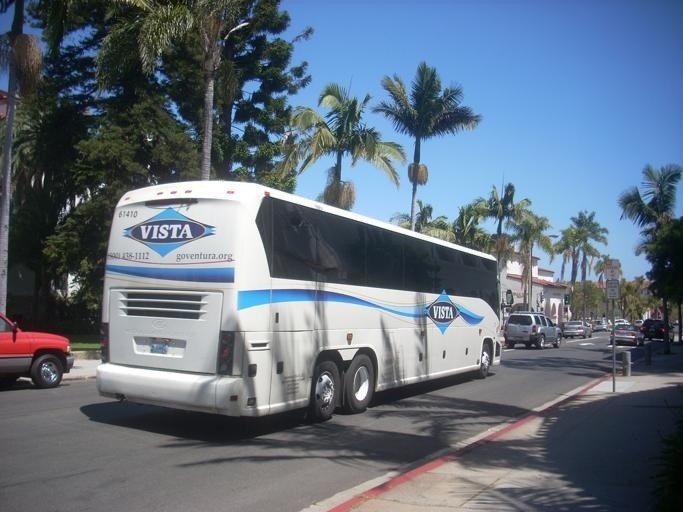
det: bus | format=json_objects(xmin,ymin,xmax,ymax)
[{"xmin": 96, "ymin": 180, "xmax": 505, "ymax": 421}]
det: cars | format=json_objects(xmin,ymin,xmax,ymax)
[
  {"xmin": 558, "ymin": 318, "xmax": 645, "ymax": 347},
  {"xmin": 0, "ymin": 312, "xmax": 74, "ymax": 389}
]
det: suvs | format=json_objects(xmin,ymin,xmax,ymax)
[
  {"xmin": 504, "ymin": 311, "xmax": 563, "ymax": 350},
  {"xmin": 640, "ymin": 319, "xmax": 675, "ymax": 343}
]
